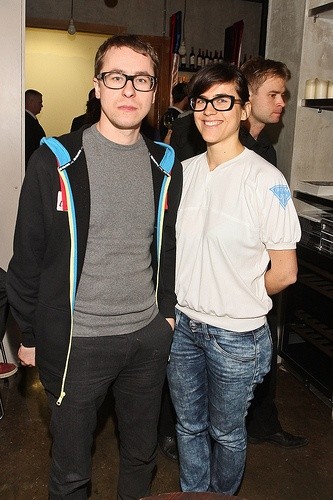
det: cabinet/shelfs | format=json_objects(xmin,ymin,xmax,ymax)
[{"xmin": 274, "ymin": 242, "xmax": 333, "ymax": 407}]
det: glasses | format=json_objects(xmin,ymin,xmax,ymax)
[
  {"xmin": 96, "ymin": 72, "xmax": 157, "ymax": 92},
  {"xmin": 188, "ymin": 95, "xmax": 242, "ymax": 112}
]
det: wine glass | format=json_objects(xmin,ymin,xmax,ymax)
[{"xmin": 163, "ymin": 113, "xmax": 174, "ymax": 132}]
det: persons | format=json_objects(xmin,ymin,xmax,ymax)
[
  {"xmin": 162, "ymin": 58, "xmax": 307, "ymax": 460},
  {"xmin": 70, "ymin": 88, "xmax": 101, "ymax": 135},
  {"xmin": 160, "ymin": 82, "xmax": 191, "ymax": 137},
  {"xmin": 7, "ymin": 36, "xmax": 182, "ymax": 500},
  {"xmin": 168, "ymin": 59, "xmax": 300, "ymax": 495},
  {"xmin": 25, "ymin": 89, "xmax": 45, "ymax": 171}
]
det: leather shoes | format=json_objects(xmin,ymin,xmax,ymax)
[
  {"xmin": 246, "ymin": 429, "xmax": 308, "ymax": 448},
  {"xmin": 158, "ymin": 433, "xmax": 179, "ymax": 463}
]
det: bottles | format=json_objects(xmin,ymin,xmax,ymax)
[
  {"xmin": 202, "ymin": 51, "xmax": 204, "ymax": 68},
  {"xmin": 205, "ymin": 49, "xmax": 209, "ymax": 66},
  {"xmin": 210, "ymin": 52, "xmax": 213, "ymax": 63},
  {"xmin": 180, "ymin": 54, "xmax": 187, "ymax": 67},
  {"xmin": 213, "ymin": 50, "xmax": 218, "ymax": 63},
  {"xmin": 190, "ymin": 47, "xmax": 195, "ymax": 69},
  {"xmin": 197, "ymin": 49, "xmax": 202, "ymax": 68},
  {"xmin": 218, "ymin": 50, "xmax": 224, "ymax": 63}
]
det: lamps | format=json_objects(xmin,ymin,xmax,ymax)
[
  {"xmin": 68, "ymin": 0, "xmax": 76, "ymax": 35},
  {"xmin": 178, "ymin": 0, "xmax": 187, "ymax": 55}
]
